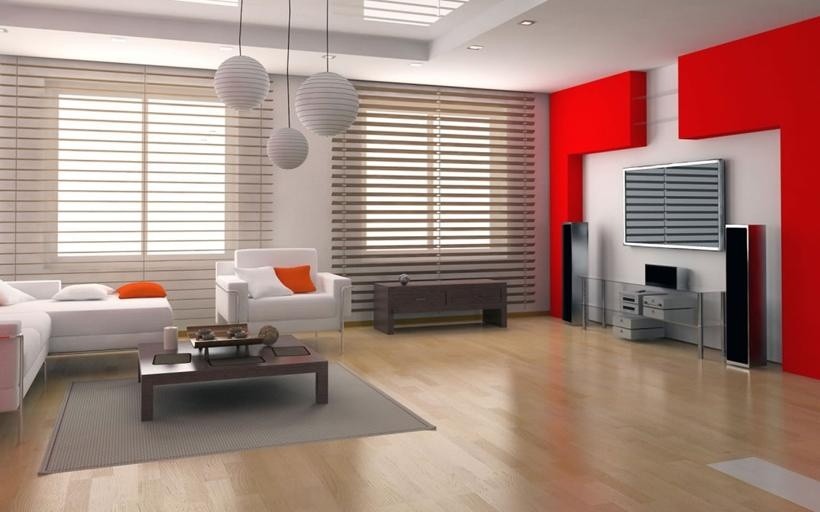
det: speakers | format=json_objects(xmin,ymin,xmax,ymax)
[
  {"xmin": 562, "ymin": 220, "xmax": 589, "ymax": 324},
  {"xmin": 721, "ymin": 224, "xmax": 767, "ymax": 370}
]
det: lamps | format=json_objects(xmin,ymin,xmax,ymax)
[
  {"xmin": 294, "ymin": 0, "xmax": 359, "ymax": 139},
  {"xmin": 213, "ymin": 0, "xmax": 270, "ymax": 113},
  {"xmin": 266, "ymin": 0, "xmax": 309, "ymax": 170}
]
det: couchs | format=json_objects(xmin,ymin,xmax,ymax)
[{"xmin": 1, "ymin": 279, "xmax": 175, "ymax": 445}]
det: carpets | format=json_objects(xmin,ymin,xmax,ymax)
[{"xmin": 36, "ymin": 360, "xmax": 437, "ymax": 477}]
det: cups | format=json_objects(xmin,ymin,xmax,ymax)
[{"xmin": 163, "ymin": 326, "xmax": 178, "ymax": 351}]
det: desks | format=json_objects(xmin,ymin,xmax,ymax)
[{"xmin": 373, "ymin": 278, "xmax": 508, "ymax": 335}]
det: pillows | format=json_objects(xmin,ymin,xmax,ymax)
[
  {"xmin": 234, "ymin": 266, "xmax": 295, "ymax": 300},
  {"xmin": 116, "ymin": 283, "xmax": 167, "ymax": 300},
  {"xmin": 233, "ymin": 246, "xmax": 318, "ymax": 296},
  {"xmin": 274, "ymin": 265, "xmax": 317, "ymax": 294},
  {"xmin": 1, "ymin": 280, "xmax": 34, "ymax": 306},
  {"xmin": 52, "ymin": 283, "xmax": 116, "ymax": 301}
]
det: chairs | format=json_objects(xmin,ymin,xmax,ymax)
[{"xmin": 214, "ymin": 247, "xmax": 352, "ymax": 355}]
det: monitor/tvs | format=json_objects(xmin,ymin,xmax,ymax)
[{"xmin": 622, "ymin": 159, "xmax": 727, "ymax": 253}]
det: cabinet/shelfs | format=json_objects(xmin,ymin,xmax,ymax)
[{"xmin": 576, "ymin": 273, "xmax": 725, "ymax": 361}]
